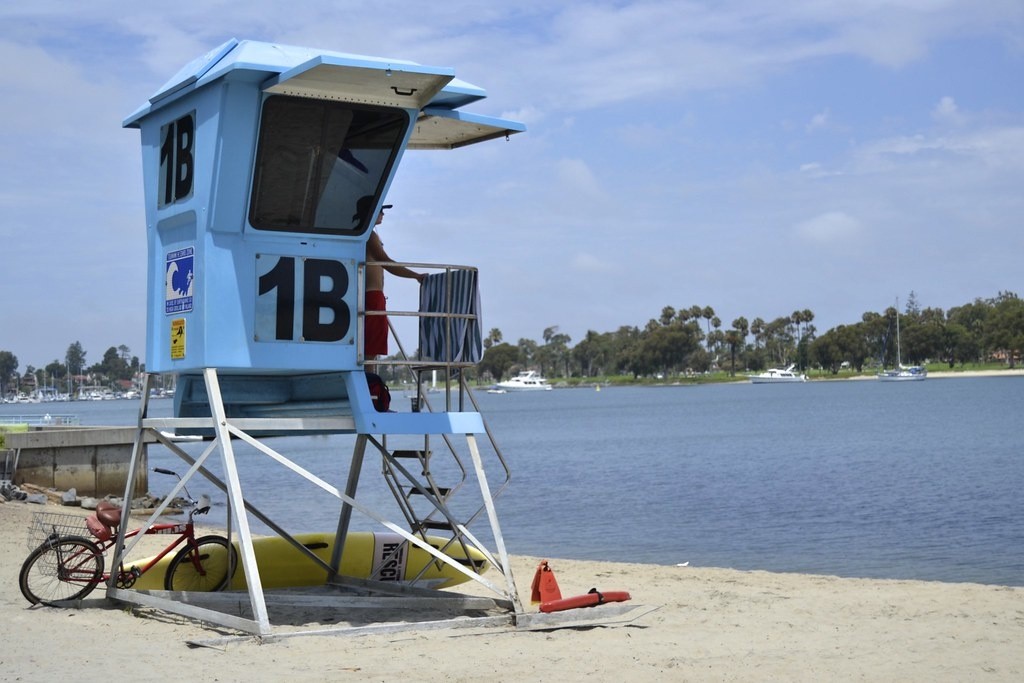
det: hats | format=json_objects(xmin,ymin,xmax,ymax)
[{"xmin": 357, "ymin": 195, "xmax": 393, "ymax": 213}]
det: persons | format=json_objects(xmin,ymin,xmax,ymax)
[{"xmin": 352, "ymin": 196, "xmax": 429, "ymax": 373}]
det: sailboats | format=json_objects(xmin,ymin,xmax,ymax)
[
  {"xmin": 876, "ymin": 296, "xmax": 927, "ymax": 381},
  {"xmin": 0, "ymin": 366, "xmax": 175, "ymax": 405}
]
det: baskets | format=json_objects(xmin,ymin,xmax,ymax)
[
  {"xmin": 27, "ymin": 513, "xmax": 90, "ymax": 553},
  {"xmin": 37, "ymin": 532, "xmax": 107, "ymax": 579}
]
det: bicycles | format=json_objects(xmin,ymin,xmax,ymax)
[{"xmin": 19, "ymin": 468, "xmax": 237, "ymax": 608}]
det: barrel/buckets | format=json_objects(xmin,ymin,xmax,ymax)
[{"xmin": 411, "ymin": 398, "xmax": 419, "ymax": 412}]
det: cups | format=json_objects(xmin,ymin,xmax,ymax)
[{"xmin": 197, "ymin": 494, "xmax": 210, "ymax": 510}]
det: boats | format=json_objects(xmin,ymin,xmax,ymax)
[
  {"xmin": 427, "ymin": 388, "xmax": 439, "ymax": 394},
  {"xmin": 749, "ymin": 369, "xmax": 805, "ymax": 385},
  {"xmin": 487, "ymin": 389, "xmax": 506, "ymax": 394},
  {"xmin": 496, "ymin": 370, "xmax": 553, "ymax": 391}
]
